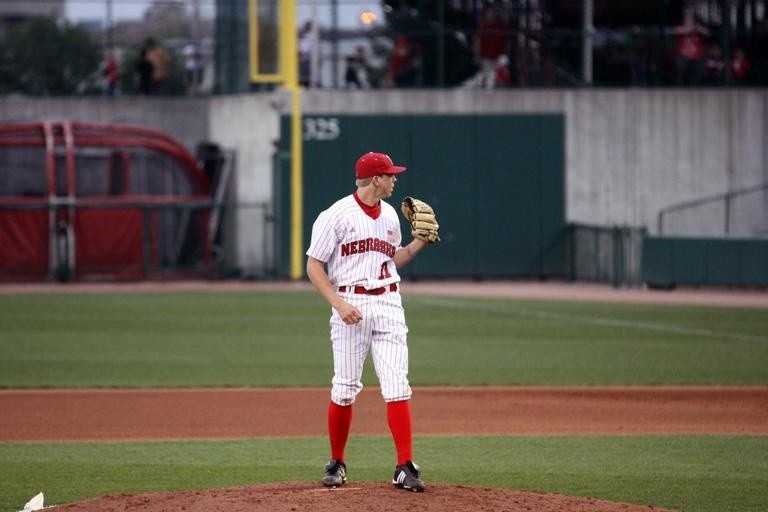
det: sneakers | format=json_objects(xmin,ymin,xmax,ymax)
[
  {"xmin": 320, "ymin": 458, "xmax": 349, "ymax": 488},
  {"xmin": 391, "ymin": 459, "xmax": 426, "ymax": 493}
]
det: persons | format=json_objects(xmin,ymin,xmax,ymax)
[
  {"xmin": 92, "ymin": 0, "xmax": 767, "ymax": 95},
  {"xmin": 306, "ymin": 153, "xmax": 441, "ymax": 495}
]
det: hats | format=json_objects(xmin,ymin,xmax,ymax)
[{"xmin": 354, "ymin": 150, "xmax": 408, "ymax": 179}]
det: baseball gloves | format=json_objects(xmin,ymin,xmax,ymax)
[{"xmin": 401, "ymin": 196, "xmax": 439, "ymax": 244}]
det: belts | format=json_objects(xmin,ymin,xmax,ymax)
[{"xmin": 338, "ymin": 282, "xmax": 399, "ymax": 296}]
